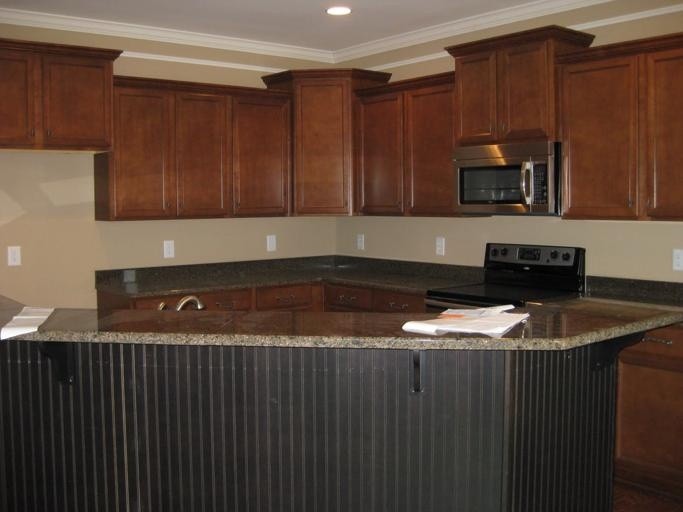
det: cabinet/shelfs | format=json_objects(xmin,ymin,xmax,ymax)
[
  {"xmin": 355, "ymin": 69, "xmax": 456, "ymax": 217},
  {"xmin": 565, "ymin": 313, "xmax": 683, "ymax": 357},
  {"xmin": 94, "ymin": 284, "xmax": 428, "ymax": 316},
  {"xmin": 0, "ymin": 37, "xmax": 123, "ymax": 152},
  {"xmin": 234, "ymin": 68, "xmax": 392, "ymax": 218},
  {"xmin": 93, "ymin": 75, "xmax": 234, "ymax": 221},
  {"xmin": 559, "ymin": 29, "xmax": 683, "ymax": 222},
  {"xmin": 442, "ymin": 22, "xmax": 597, "ymax": 145}
]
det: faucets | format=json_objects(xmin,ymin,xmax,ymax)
[{"xmin": 176, "ymin": 296, "xmax": 204, "ymax": 311}]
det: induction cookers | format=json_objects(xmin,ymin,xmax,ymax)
[{"xmin": 431, "ymin": 281, "xmax": 581, "ymax": 302}]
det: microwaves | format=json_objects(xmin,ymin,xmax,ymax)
[{"xmin": 448, "ymin": 136, "xmax": 560, "ymax": 218}]
